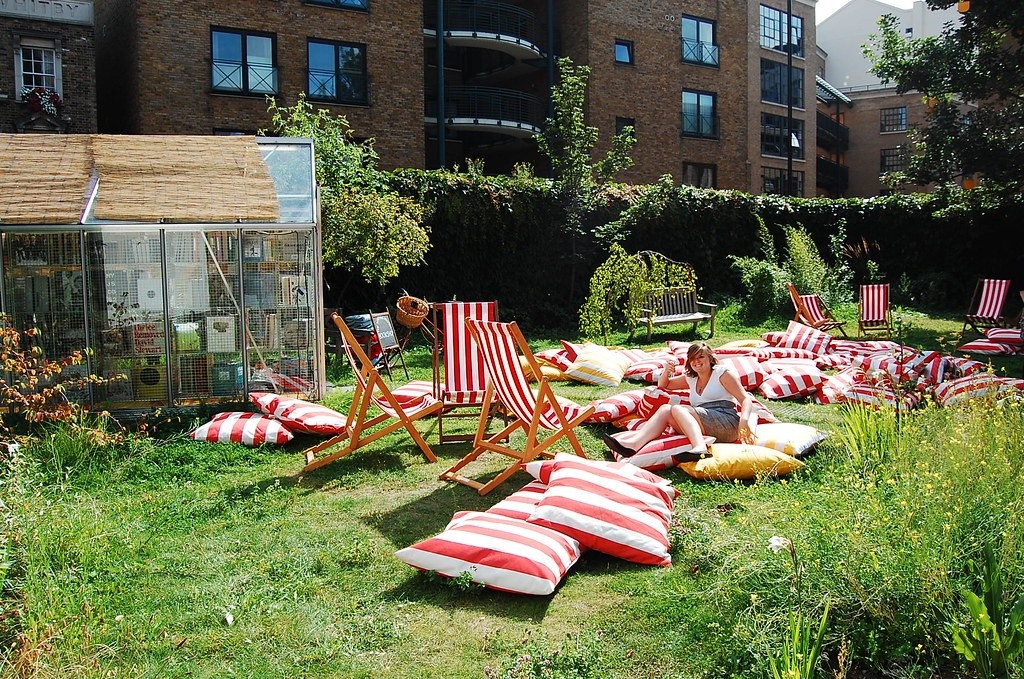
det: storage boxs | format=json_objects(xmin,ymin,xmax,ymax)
[{"xmin": 101, "ymin": 273, "xmax": 318, "ymax": 403}]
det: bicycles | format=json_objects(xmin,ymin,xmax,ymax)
[{"xmin": 382, "ymin": 288, "xmax": 444, "ymax": 371}]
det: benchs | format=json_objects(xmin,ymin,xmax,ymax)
[{"xmin": 624, "ymin": 250, "xmax": 716, "ymax": 343}]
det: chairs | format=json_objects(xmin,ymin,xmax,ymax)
[
  {"xmin": 301, "ymin": 311, "xmax": 443, "ymax": 474},
  {"xmin": 440, "ymin": 318, "xmax": 596, "ymax": 495},
  {"xmin": 961, "ymin": 278, "xmax": 1013, "ymax": 338},
  {"xmin": 857, "ymin": 282, "xmax": 891, "ymax": 339},
  {"xmin": 433, "ymin": 299, "xmax": 511, "ymax": 446},
  {"xmin": 786, "ymin": 282, "xmax": 848, "ymax": 339}
]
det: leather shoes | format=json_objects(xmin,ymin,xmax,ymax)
[
  {"xmin": 603, "ymin": 433, "xmax": 635, "ymax": 458},
  {"xmin": 674, "ymin": 450, "xmax": 712, "ymax": 462}
]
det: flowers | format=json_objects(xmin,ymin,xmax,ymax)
[{"xmin": 20, "ymin": 85, "xmax": 62, "ymax": 118}]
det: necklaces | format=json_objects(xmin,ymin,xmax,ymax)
[{"xmin": 699, "ymin": 387, "xmax": 703, "ymax": 393}]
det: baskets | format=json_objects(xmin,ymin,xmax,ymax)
[{"xmin": 395, "ymin": 297, "xmax": 429, "ymax": 329}]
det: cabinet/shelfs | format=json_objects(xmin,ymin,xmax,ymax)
[{"xmin": 5, "ymin": 262, "xmax": 313, "ymax": 395}]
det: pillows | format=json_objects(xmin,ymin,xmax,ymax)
[{"xmin": 193, "ymin": 275, "xmax": 1023, "ymax": 598}]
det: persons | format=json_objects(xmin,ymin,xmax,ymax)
[{"xmin": 603, "ymin": 342, "xmax": 758, "ymax": 462}]
